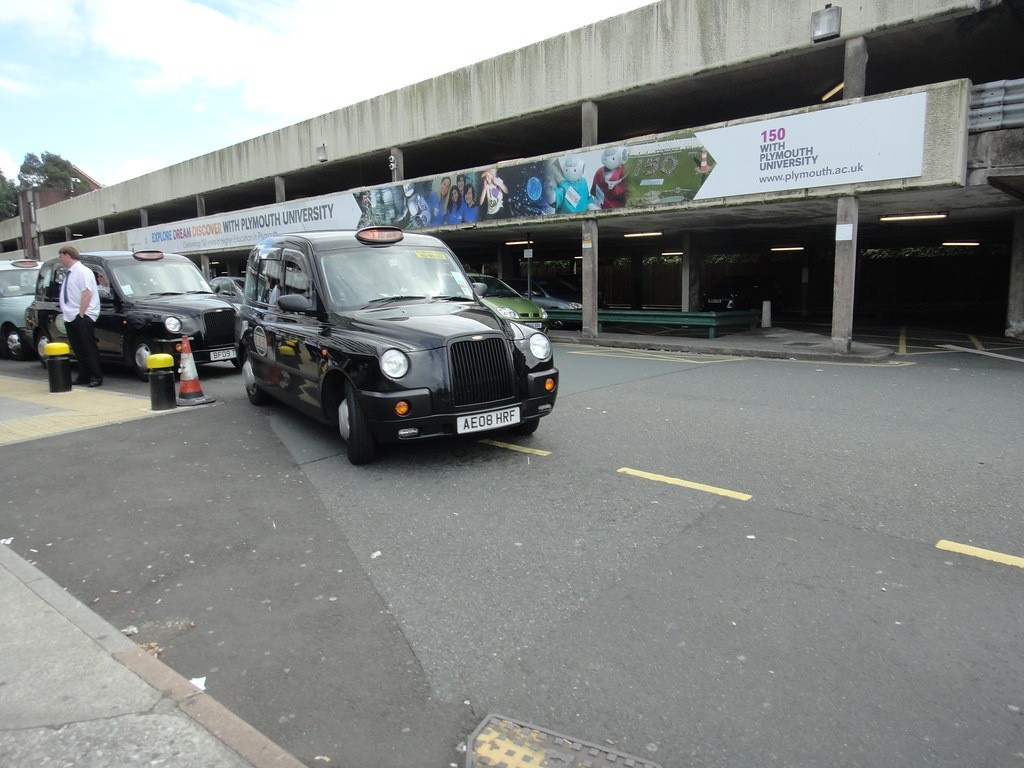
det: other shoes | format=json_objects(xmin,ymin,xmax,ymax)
[
  {"xmin": 71, "ymin": 379, "xmax": 88, "ymax": 385},
  {"xmin": 89, "ymin": 380, "xmax": 102, "ymax": 387}
]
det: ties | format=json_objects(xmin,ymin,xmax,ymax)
[{"xmin": 63, "ymin": 270, "xmax": 71, "ymax": 304}]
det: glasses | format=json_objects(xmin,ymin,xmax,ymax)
[{"xmin": 96, "ymin": 275, "xmax": 103, "ymax": 278}]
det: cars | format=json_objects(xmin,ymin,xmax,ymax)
[
  {"xmin": 466, "ymin": 272, "xmax": 549, "ymax": 336},
  {"xmin": 500, "ymin": 276, "xmax": 583, "ymax": 330},
  {"xmin": 209, "ymin": 276, "xmax": 247, "ymax": 304},
  {"xmin": 0, "ymin": 258, "xmax": 51, "ymax": 362},
  {"xmin": 24, "ymin": 249, "xmax": 237, "ymax": 382},
  {"xmin": 234, "ymin": 226, "xmax": 558, "ymax": 465}
]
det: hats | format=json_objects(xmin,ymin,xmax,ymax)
[
  {"xmin": 402, "ymin": 183, "xmax": 415, "ymax": 197},
  {"xmin": 481, "ymin": 169, "xmax": 498, "ymax": 178}
]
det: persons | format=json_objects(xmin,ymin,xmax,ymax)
[
  {"xmin": 97, "ymin": 273, "xmax": 110, "ymax": 294},
  {"xmin": 58, "ymin": 246, "xmax": 104, "ymax": 387},
  {"xmin": 426, "ymin": 168, "xmax": 509, "ymax": 227}
]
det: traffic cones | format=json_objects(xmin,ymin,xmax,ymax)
[{"xmin": 174, "ymin": 335, "xmax": 216, "ymax": 407}]
